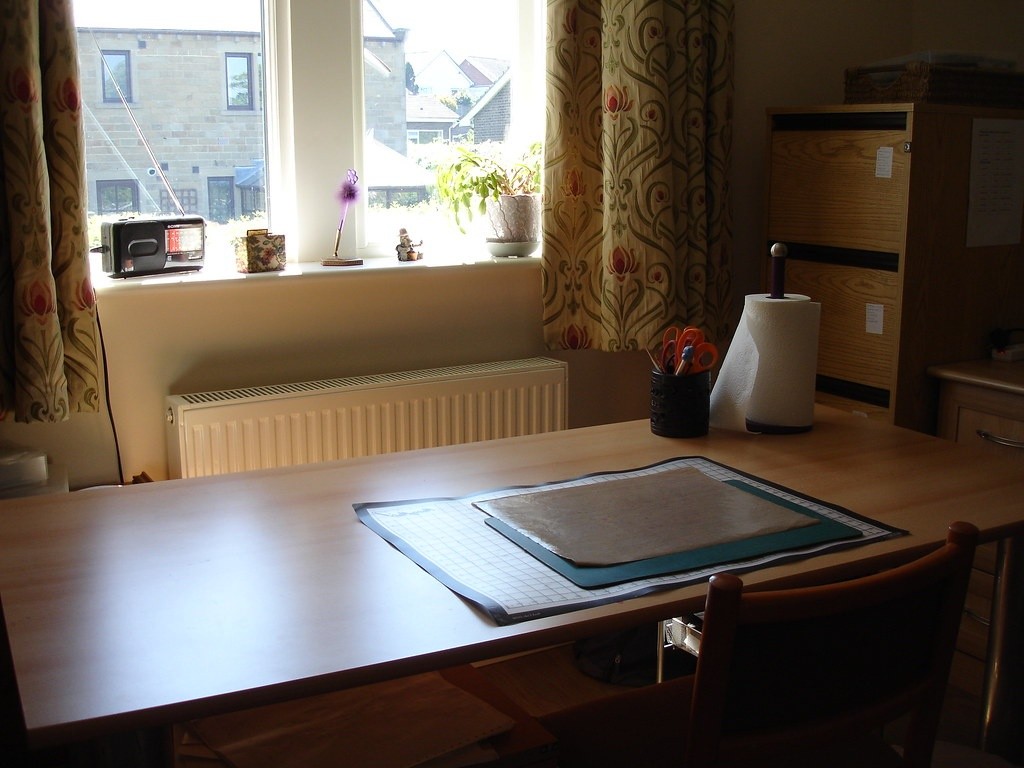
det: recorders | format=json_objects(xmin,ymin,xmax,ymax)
[{"xmin": 90, "ymin": 29, "xmax": 208, "ymax": 279}]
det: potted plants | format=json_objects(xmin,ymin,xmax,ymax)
[{"xmin": 435, "ymin": 141, "xmax": 546, "ymax": 257}]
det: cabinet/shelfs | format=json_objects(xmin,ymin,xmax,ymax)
[
  {"xmin": 929, "ymin": 359, "xmax": 1024, "ymax": 662},
  {"xmin": 762, "ymin": 103, "xmax": 1024, "ymax": 435}
]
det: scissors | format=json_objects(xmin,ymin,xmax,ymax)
[{"xmin": 661, "ymin": 325, "xmax": 718, "ymax": 374}]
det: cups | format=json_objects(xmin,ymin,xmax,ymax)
[{"xmin": 649, "ymin": 368, "xmax": 711, "ymax": 439}]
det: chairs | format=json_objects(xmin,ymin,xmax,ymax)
[{"xmin": 534, "ymin": 519, "xmax": 978, "ymax": 768}]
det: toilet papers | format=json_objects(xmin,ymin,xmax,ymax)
[{"xmin": 710, "ymin": 296, "xmax": 819, "ymax": 434}]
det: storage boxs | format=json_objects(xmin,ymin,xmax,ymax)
[{"xmin": 234, "ymin": 229, "xmax": 286, "ymax": 273}]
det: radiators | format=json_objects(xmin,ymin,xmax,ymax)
[{"xmin": 164, "ymin": 358, "xmax": 567, "ymax": 479}]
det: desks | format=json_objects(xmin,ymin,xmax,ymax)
[{"xmin": 0, "ymin": 405, "xmax": 1024, "ymax": 768}]
